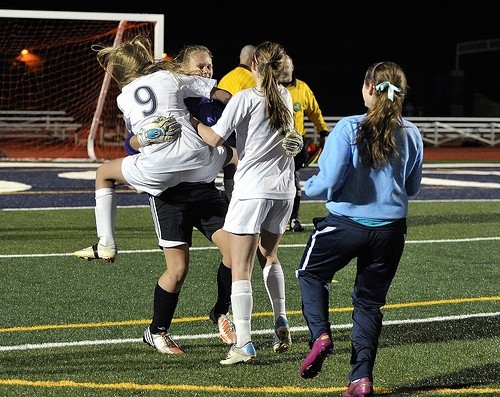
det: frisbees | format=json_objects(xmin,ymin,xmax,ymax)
[{"xmin": 304, "ymin": 147, "xmax": 322, "ymax": 168}]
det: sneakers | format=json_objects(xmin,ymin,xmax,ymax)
[
  {"xmin": 302, "ymin": 333, "xmax": 334, "ymax": 379},
  {"xmin": 143, "ymin": 326, "xmax": 185, "ymax": 355},
  {"xmin": 209, "ymin": 306, "xmax": 240, "ymax": 345},
  {"xmin": 74, "ymin": 242, "xmax": 117, "ymax": 263},
  {"xmin": 289, "ymin": 218, "xmax": 305, "ymax": 232},
  {"xmin": 219, "ymin": 342, "xmax": 256, "ymax": 365},
  {"xmin": 273, "ymin": 316, "xmax": 292, "ymax": 352},
  {"xmin": 342, "ymin": 376, "xmax": 373, "ymax": 397}
]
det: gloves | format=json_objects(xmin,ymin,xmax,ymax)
[
  {"xmin": 136, "ymin": 115, "xmax": 182, "ymax": 146},
  {"xmin": 319, "ymin": 130, "xmax": 329, "ymax": 148},
  {"xmin": 282, "ymin": 130, "xmax": 304, "ymax": 156}
]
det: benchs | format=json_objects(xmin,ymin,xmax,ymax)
[{"xmin": 0, "ymin": 111, "xmax": 82, "ymax": 144}]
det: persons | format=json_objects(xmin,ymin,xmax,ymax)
[
  {"xmin": 70, "ymin": 37, "xmax": 232, "ymax": 263},
  {"xmin": 216, "ymin": 45, "xmax": 330, "ymax": 236},
  {"xmin": 188, "ymin": 42, "xmax": 295, "ymax": 366},
  {"xmin": 294, "ymin": 62, "xmax": 424, "ymax": 397},
  {"xmin": 124, "ymin": 45, "xmax": 238, "ymax": 355}
]
